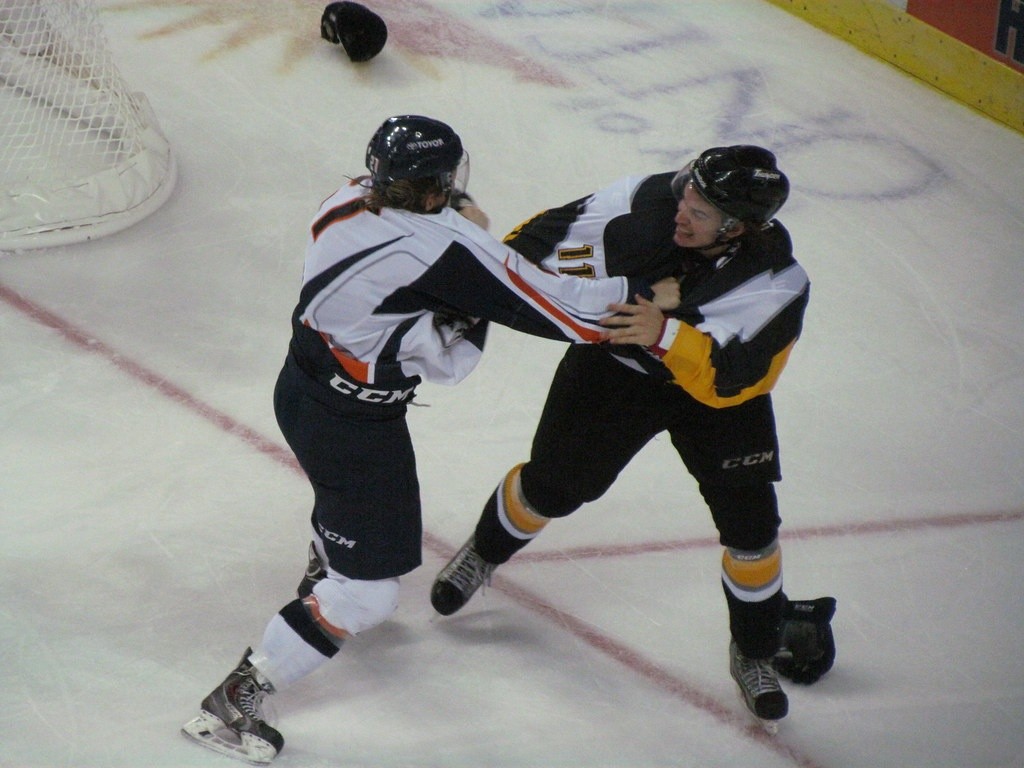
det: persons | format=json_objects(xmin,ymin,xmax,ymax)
[
  {"xmin": 180, "ymin": 117, "xmax": 681, "ymax": 764},
  {"xmin": 429, "ymin": 145, "xmax": 810, "ymax": 721}
]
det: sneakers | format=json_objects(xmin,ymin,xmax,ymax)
[
  {"xmin": 181, "ymin": 647, "xmax": 284, "ymax": 767},
  {"xmin": 430, "ymin": 532, "xmax": 499, "ymax": 622},
  {"xmin": 261, "ymin": 540, "xmax": 328, "ymax": 634},
  {"xmin": 730, "ymin": 636, "xmax": 789, "ymax": 739}
]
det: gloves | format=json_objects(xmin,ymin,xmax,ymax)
[
  {"xmin": 321, "ymin": 1, "xmax": 387, "ymax": 63},
  {"xmin": 771, "ymin": 597, "xmax": 836, "ymax": 686}
]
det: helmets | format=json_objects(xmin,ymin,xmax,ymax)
[
  {"xmin": 691, "ymin": 144, "xmax": 789, "ymax": 229},
  {"xmin": 366, "ymin": 115, "xmax": 463, "ymax": 192}
]
